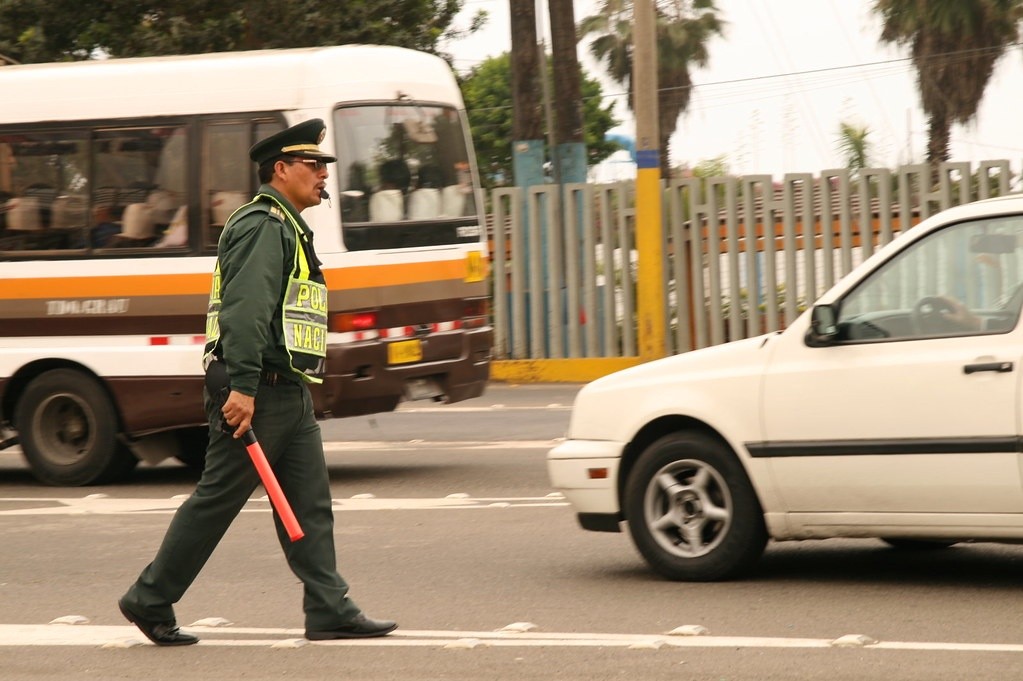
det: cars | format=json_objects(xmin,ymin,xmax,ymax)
[{"xmin": 545, "ymin": 193, "xmax": 1022, "ymax": 582}]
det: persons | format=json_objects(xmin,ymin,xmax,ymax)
[
  {"xmin": 937, "ymin": 294, "xmax": 1011, "ymax": 331},
  {"xmin": 117, "ymin": 118, "xmax": 400, "ymax": 646},
  {"xmin": 416, "ymin": 165, "xmax": 447, "ymax": 188},
  {"xmin": 91, "ymin": 208, "xmax": 121, "ymax": 248},
  {"xmin": 377, "ymin": 160, "xmax": 410, "ymax": 193}
]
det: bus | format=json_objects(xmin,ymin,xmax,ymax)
[{"xmin": 0, "ymin": 41, "xmax": 498, "ymax": 487}]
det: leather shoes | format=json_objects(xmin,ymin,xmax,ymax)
[
  {"xmin": 305, "ymin": 611, "xmax": 399, "ymax": 640},
  {"xmin": 119, "ymin": 599, "xmax": 200, "ymax": 645}
]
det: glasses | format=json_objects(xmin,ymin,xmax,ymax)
[{"xmin": 272, "ymin": 159, "xmax": 327, "ymax": 171}]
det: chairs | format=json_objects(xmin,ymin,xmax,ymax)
[{"xmin": 2, "ymin": 185, "xmax": 469, "ymax": 252}]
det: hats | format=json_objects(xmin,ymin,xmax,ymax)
[{"xmin": 248, "ymin": 118, "xmax": 337, "ymax": 165}]
